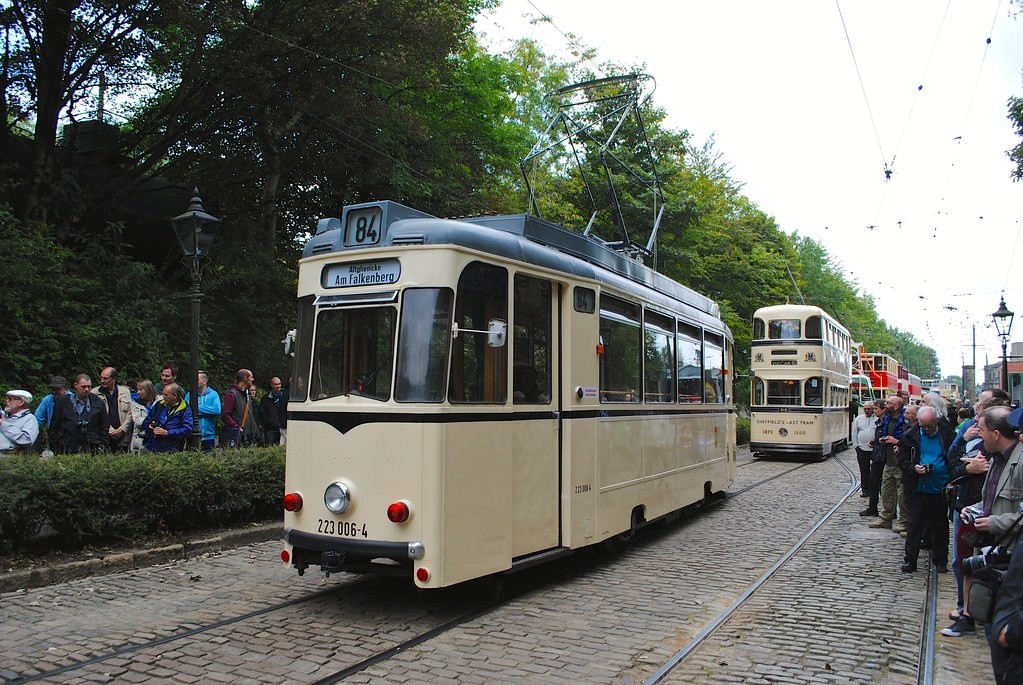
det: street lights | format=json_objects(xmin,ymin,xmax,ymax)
[
  {"xmin": 169, "ymin": 184, "xmax": 223, "ymax": 447},
  {"xmin": 991, "ymin": 296, "xmax": 1015, "ymax": 392}
]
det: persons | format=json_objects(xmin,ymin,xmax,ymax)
[
  {"xmin": 260, "ymin": 376, "xmax": 283, "ymax": 446},
  {"xmin": 279, "ymin": 377, "xmax": 303, "ymax": 446},
  {"xmin": 739, "ymin": 406, "xmax": 749, "ymax": 418},
  {"xmin": 768, "ymin": 382, "xmax": 784, "ymax": 404},
  {"xmin": 132, "ymin": 378, "xmax": 164, "ymax": 454},
  {"xmin": 34, "ymin": 376, "xmax": 74, "ymax": 432},
  {"xmin": 692, "ymin": 372, "xmax": 716, "ymax": 403},
  {"xmin": 47, "ymin": 374, "xmax": 108, "ymax": 455},
  {"xmin": 605, "ymin": 366, "xmax": 631, "ymax": 402},
  {"xmin": 250, "ymin": 384, "xmax": 259, "ymax": 414},
  {"xmin": 0, "ymin": 390, "xmax": 40, "ymax": 453},
  {"xmin": 184, "ymin": 370, "xmax": 221, "ymax": 450},
  {"xmin": 221, "ymin": 369, "xmax": 264, "ymax": 448},
  {"xmin": 141, "ymin": 384, "xmax": 193, "ymax": 454},
  {"xmin": 849, "ymin": 388, "xmax": 1023, "ymax": 685},
  {"xmin": 807, "ymin": 384, "xmax": 821, "ymax": 404},
  {"xmin": 89, "ymin": 367, "xmax": 134, "ymax": 454},
  {"xmin": 121, "ymin": 375, "xmax": 139, "ymax": 401},
  {"xmin": 154, "ymin": 364, "xmax": 185, "ymax": 400},
  {"xmin": 513, "ymin": 363, "xmax": 548, "ymax": 402}
]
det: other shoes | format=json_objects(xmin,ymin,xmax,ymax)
[
  {"xmin": 868, "ymin": 517, "xmax": 892, "ymax": 529},
  {"xmin": 937, "ymin": 563, "xmax": 948, "ymax": 572},
  {"xmin": 893, "ymin": 524, "xmax": 907, "ymax": 532},
  {"xmin": 949, "ymin": 607, "xmax": 963, "ymax": 619},
  {"xmin": 860, "ymin": 508, "xmax": 878, "ymax": 516},
  {"xmin": 902, "ymin": 561, "xmax": 918, "ymax": 572},
  {"xmin": 899, "ymin": 531, "xmax": 908, "ymax": 538},
  {"xmin": 860, "ymin": 490, "xmax": 869, "ymax": 497}
]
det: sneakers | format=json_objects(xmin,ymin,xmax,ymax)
[{"xmin": 941, "ymin": 615, "xmax": 975, "ymax": 637}]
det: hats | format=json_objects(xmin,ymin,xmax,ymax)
[
  {"xmin": 853, "ymin": 395, "xmax": 859, "ymax": 399},
  {"xmin": 902, "ymin": 392, "xmax": 910, "ymax": 397},
  {"xmin": 6, "ymin": 390, "xmax": 33, "ymax": 404},
  {"xmin": 1006, "ymin": 406, "xmax": 1023, "ymax": 429},
  {"xmin": 47, "ymin": 376, "xmax": 67, "ymax": 388}
]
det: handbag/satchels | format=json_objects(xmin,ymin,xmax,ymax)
[{"xmin": 967, "ymin": 569, "xmax": 1005, "ymax": 625}]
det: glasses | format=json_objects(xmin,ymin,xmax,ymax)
[
  {"xmin": 8, "ymin": 397, "xmax": 21, "ymax": 401},
  {"xmin": 919, "ymin": 421, "xmax": 936, "ymax": 428},
  {"xmin": 887, "ymin": 400, "xmax": 894, "ymax": 405},
  {"xmin": 954, "ymin": 403, "xmax": 959, "ymax": 405}
]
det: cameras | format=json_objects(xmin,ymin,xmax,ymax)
[
  {"xmin": 76, "ymin": 420, "xmax": 89, "ymax": 434},
  {"xmin": 962, "ymin": 506, "xmax": 985, "ymax": 525},
  {"xmin": 893, "ymin": 448, "xmax": 898, "ymax": 454},
  {"xmin": 962, "ymin": 545, "xmax": 1013, "ymax": 576},
  {"xmin": 922, "ymin": 463, "xmax": 933, "ymax": 472},
  {"xmin": 882, "ymin": 436, "xmax": 887, "ymax": 442},
  {"xmin": 150, "ymin": 418, "xmax": 160, "ymax": 428}
]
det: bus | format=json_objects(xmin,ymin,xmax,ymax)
[
  {"xmin": 278, "ymin": 199, "xmax": 739, "ymax": 591},
  {"xmin": 750, "ymin": 303, "xmax": 851, "ymax": 464},
  {"xmin": 851, "ymin": 352, "xmax": 959, "ymax": 420}
]
